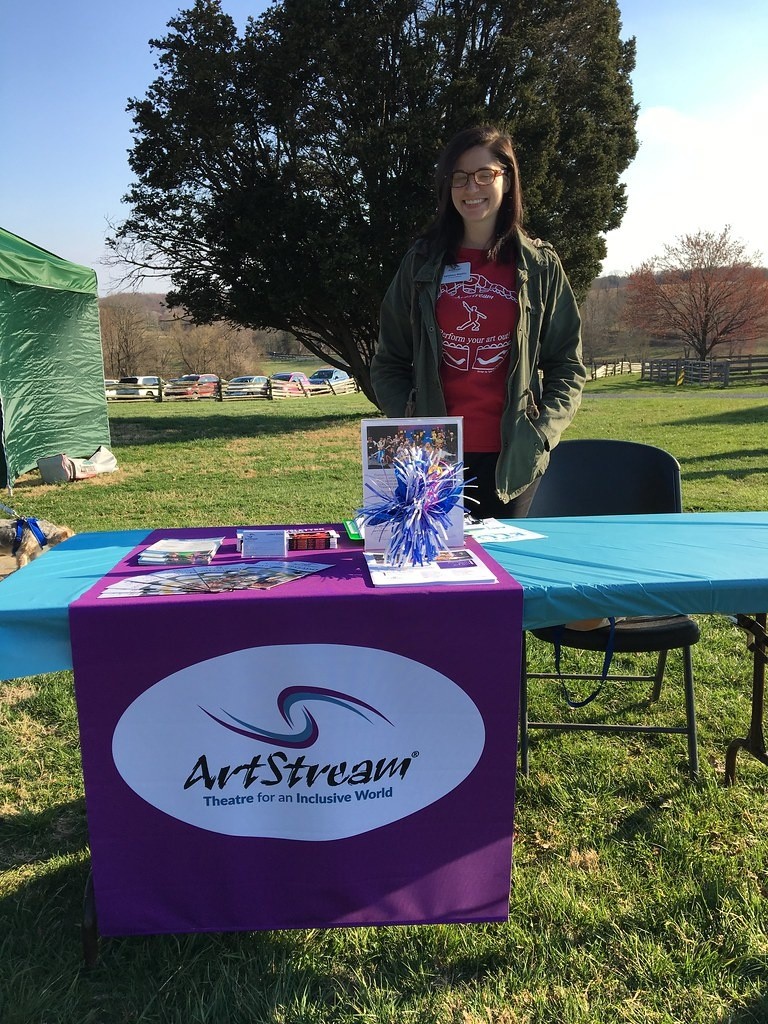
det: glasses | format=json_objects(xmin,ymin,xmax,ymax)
[{"xmin": 446, "ymin": 168, "xmax": 507, "ymax": 188}]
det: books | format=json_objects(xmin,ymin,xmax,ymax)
[
  {"xmin": 137, "ymin": 536, "xmax": 224, "ymax": 566},
  {"xmin": 96, "ymin": 560, "xmax": 338, "ymax": 600},
  {"xmin": 235, "ymin": 528, "xmax": 341, "ymax": 551}
]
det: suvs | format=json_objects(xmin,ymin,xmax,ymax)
[{"xmin": 164, "ymin": 373, "xmax": 221, "ymax": 400}]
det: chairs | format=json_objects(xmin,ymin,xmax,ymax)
[{"xmin": 518, "ymin": 438, "xmax": 705, "ymax": 782}]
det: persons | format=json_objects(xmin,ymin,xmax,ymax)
[{"xmin": 367, "ymin": 123, "xmax": 588, "ymax": 519}]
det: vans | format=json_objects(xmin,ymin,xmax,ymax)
[{"xmin": 117, "ymin": 375, "xmax": 172, "ymax": 398}]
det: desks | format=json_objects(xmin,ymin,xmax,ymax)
[{"xmin": 1, "ymin": 511, "xmax": 767, "ymax": 795}]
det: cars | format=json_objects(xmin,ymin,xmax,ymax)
[
  {"xmin": 225, "ymin": 376, "xmax": 269, "ymax": 397},
  {"xmin": 309, "ymin": 368, "xmax": 349, "ymax": 386},
  {"xmin": 104, "ymin": 380, "xmax": 118, "ymax": 400},
  {"xmin": 268, "ymin": 372, "xmax": 312, "ymax": 398}
]
